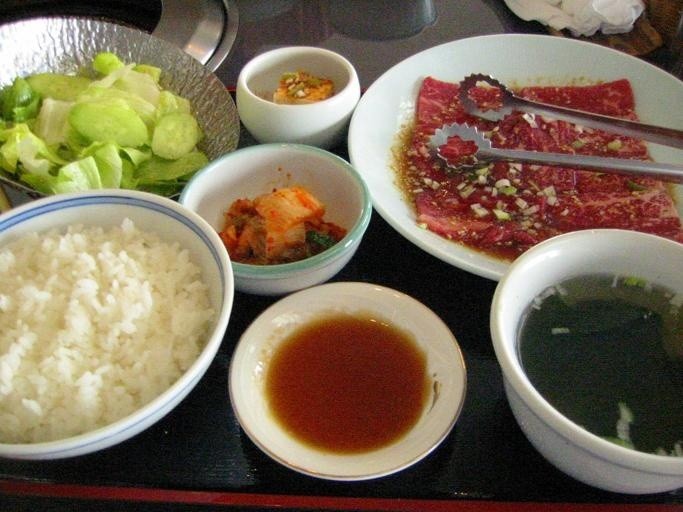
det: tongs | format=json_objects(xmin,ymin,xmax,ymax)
[{"xmin": 426, "ymin": 69, "xmax": 683, "ymax": 186}]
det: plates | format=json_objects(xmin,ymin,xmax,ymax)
[
  {"xmin": 227, "ymin": 279, "xmax": 465, "ymax": 482},
  {"xmin": 345, "ymin": 32, "xmax": 682, "ymax": 284}
]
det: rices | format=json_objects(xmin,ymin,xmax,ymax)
[{"xmin": 0, "ymin": 219, "xmax": 215, "ymax": 444}]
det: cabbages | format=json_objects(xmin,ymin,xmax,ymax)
[{"xmin": 1, "ymin": 52, "xmax": 209, "ymax": 196}]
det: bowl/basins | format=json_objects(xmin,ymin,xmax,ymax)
[
  {"xmin": 0, "ymin": 20, "xmax": 239, "ymax": 202},
  {"xmin": 173, "ymin": 144, "xmax": 370, "ymax": 300},
  {"xmin": 232, "ymin": 47, "xmax": 362, "ymax": 151},
  {"xmin": 490, "ymin": 228, "xmax": 682, "ymax": 495},
  {"xmin": 0, "ymin": 187, "xmax": 234, "ymax": 461},
  {"xmin": 324, "ymin": 0, "xmax": 441, "ymax": 42}
]
